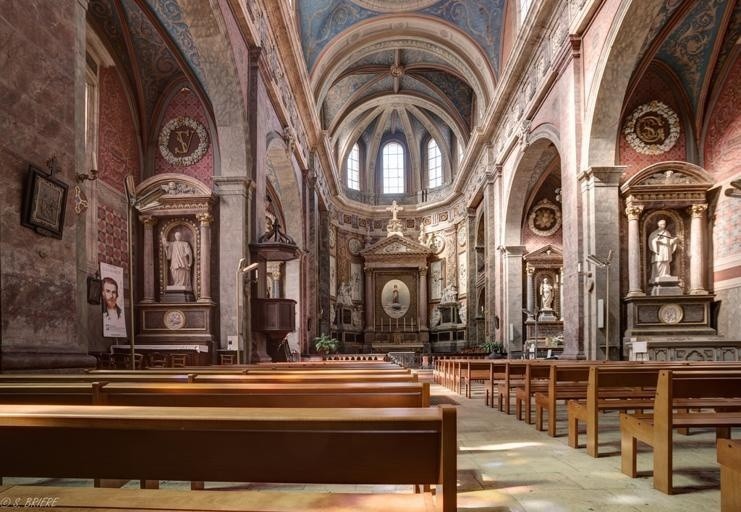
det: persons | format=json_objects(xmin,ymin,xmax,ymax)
[
  {"xmin": 539, "ymin": 277, "xmax": 555, "ymax": 310},
  {"xmin": 329, "ymin": 281, "xmax": 468, "ymax": 328},
  {"xmin": 162, "ymin": 232, "xmax": 193, "ymax": 286},
  {"xmin": 101, "ymin": 277, "xmax": 123, "ymax": 320},
  {"xmin": 647, "ymin": 219, "xmax": 680, "ymax": 279}
]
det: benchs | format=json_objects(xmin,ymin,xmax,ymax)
[
  {"xmin": 1, "ymin": 362, "xmax": 459, "ymax": 510},
  {"xmin": 431, "ymin": 353, "xmax": 740, "ymax": 510}
]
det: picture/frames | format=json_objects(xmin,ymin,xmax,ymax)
[{"xmin": 19, "ymin": 164, "xmax": 71, "ymax": 244}]
